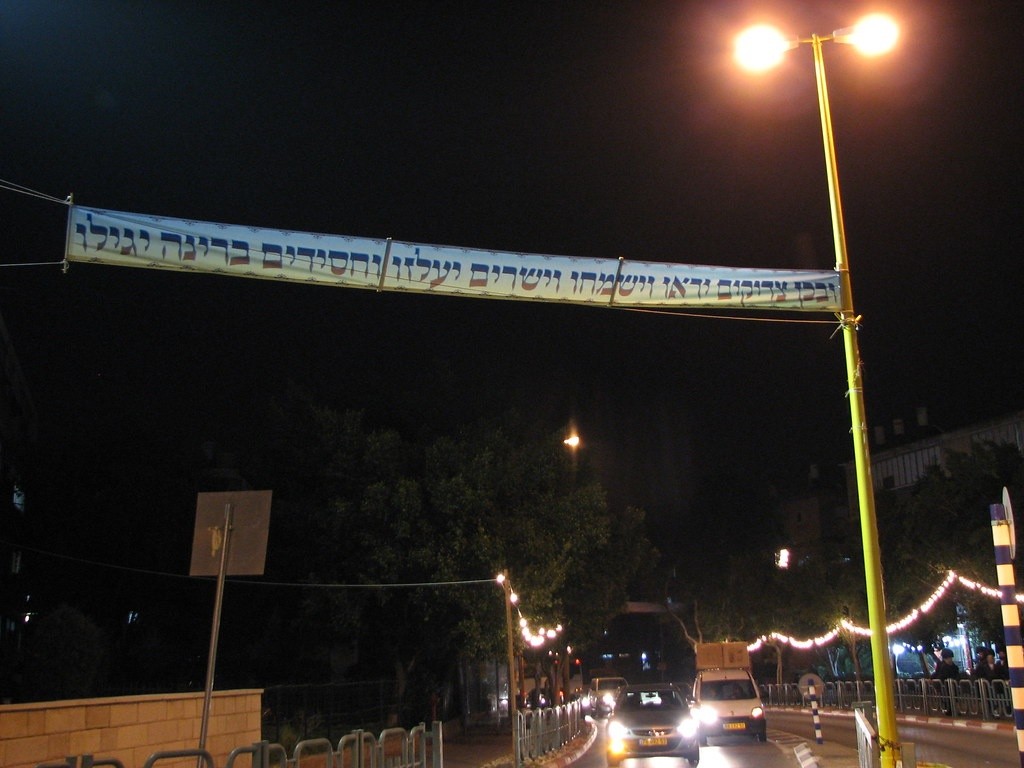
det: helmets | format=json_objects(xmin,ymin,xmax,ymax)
[
  {"xmin": 996, "ymin": 644, "xmax": 1006, "ymax": 652},
  {"xmin": 975, "ymin": 646, "xmax": 985, "ymax": 653},
  {"xmin": 984, "ymin": 649, "xmax": 995, "ymax": 657},
  {"xmin": 941, "ymin": 649, "xmax": 953, "ymax": 658}
]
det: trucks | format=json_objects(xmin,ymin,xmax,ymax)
[{"xmin": 686, "ymin": 668, "xmax": 767, "ymax": 745}]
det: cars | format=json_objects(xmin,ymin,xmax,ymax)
[
  {"xmin": 606, "ymin": 684, "xmax": 700, "ymax": 768},
  {"xmin": 526, "ymin": 688, "xmax": 550, "ymax": 710},
  {"xmin": 570, "ymin": 692, "xmax": 590, "ymax": 714}
]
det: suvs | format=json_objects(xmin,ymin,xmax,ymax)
[{"xmin": 589, "ymin": 677, "xmax": 629, "ymax": 718}]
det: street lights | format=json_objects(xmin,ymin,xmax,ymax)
[{"xmin": 731, "ymin": 13, "xmax": 905, "ymax": 768}]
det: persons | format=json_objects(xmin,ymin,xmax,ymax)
[
  {"xmin": 793, "ymin": 664, "xmax": 820, "ymax": 702},
  {"xmin": 658, "ymin": 693, "xmax": 677, "ymax": 710},
  {"xmin": 705, "ymin": 685, "xmax": 748, "ymax": 699},
  {"xmin": 973, "ymin": 646, "xmax": 1010, "ymax": 719},
  {"xmin": 936, "ymin": 649, "xmax": 960, "ymax": 716}
]
